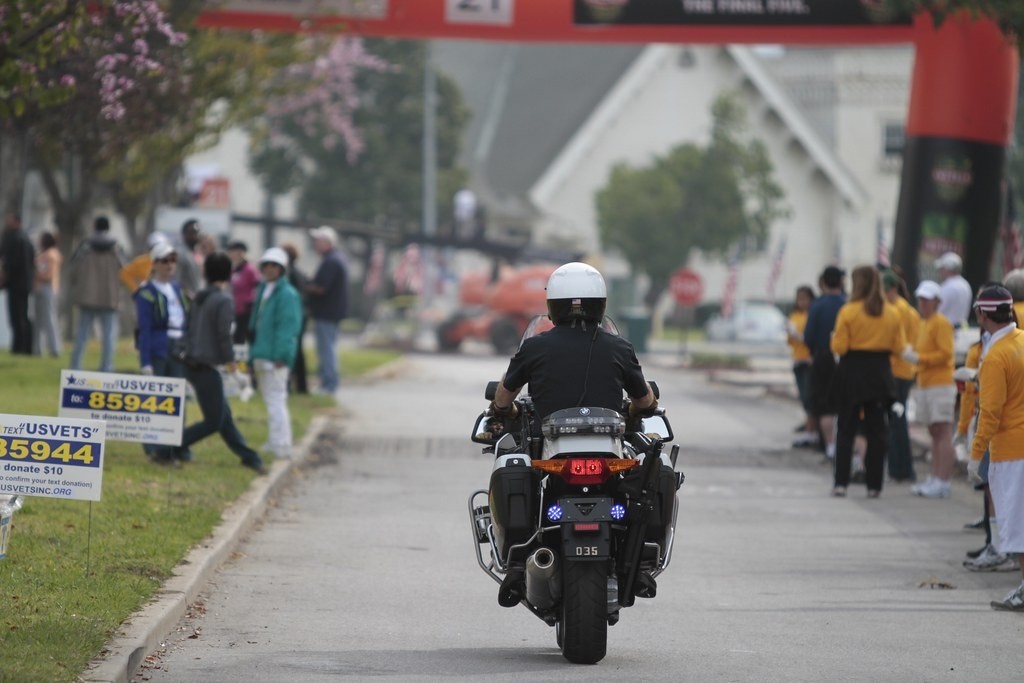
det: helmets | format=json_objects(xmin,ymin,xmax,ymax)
[{"xmin": 547, "ymin": 262, "xmax": 608, "ymax": 324}]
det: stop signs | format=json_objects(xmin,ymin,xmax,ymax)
[{"xmin": 671, "ymin": 270, "xmax": 703, "ymax": 305}]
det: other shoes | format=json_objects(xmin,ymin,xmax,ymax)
[
  {"xmin": 638, "ymin": 572, "xmax": 658, "ymax": 598},
  {"xmin": 910, "ymin": 474, "xmax": 951, "ymax": 499},
  {"xmin": 868, "ymin": 487, "xmax": 878, "ymax": 497},
  {"xmin": 245, "ymin": 459, "xmax": 268, "ymax": 475},
  {"xmin": 314, "ymin": 388, "xmax": 335, "ymax": 399},
  {"xmin": 964, "ymin": 520, "xmax": 985, "ymax": 534},
  {"xmin": 830, "ymin": 483, "xmax": 846, "ymax": 498},
  {"xmin": 263, "ymin": 439, "xmax": 292, "ymax": 456},
  {"xmin": 498, "ymin": 568, "xmax": 524, "ymax": 607}
]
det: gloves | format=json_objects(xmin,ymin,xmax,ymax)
[
  {"xmin": 628, "ymin": 395, "xmax": 658, "ymax": 420},
  {"xmin": 904, "ymin": 344, "xmax": 918, "ymax": 365},
  {"xmin": 494, "ymin": 400, "xmax": 518, "ymax": 420},
  {"xmin": 966, "ymin": 460, "xmax": 983, "ymax": 482}
]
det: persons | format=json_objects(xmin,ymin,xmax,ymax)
[
  {"xmin": 0, "ymin": 215, "xmax": 63, "ymax": 358},
  {"xmin": 130, "ymin": 197, "xmax": 352, "ymax": 475},
  {"xmin": 67, "ymin": 216, "xmax": 130, "ymax": 373},
  {"xmin": 766, "ymin": 245, "xmax": 971, "ymax": 498},
  {"xmin": 486, "ymin": 263, "xmax": 659, "ymax": 608},
  {"xmin": 948, "ymin": 267, "xmax": 1024, "ymax": 610}
]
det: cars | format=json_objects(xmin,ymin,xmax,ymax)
[{"xmin": 703, "ymin": 300, "xmax": 791, "ymax": 342}]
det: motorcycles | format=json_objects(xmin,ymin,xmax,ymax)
[
  {"xmin": 438, "ymin": 265, "xmax": 618, "ymax": 356},
  {"xmin": 470, "ymin": 379, "xmax": 686, "ymax": 663}
]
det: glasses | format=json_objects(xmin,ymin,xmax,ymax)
[{"xmin": 157, "ymin": 259, "xmax": 177, "ymax": 264}]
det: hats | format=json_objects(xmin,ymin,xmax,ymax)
[
  {"xmin": 1001, "ymin": 268, "xmax": 1024, "ymax": 301},
  {"xmin": 259, "ymin": 247, "xmax": 289, "ymax": 270},
  {"xmin": 974, "ymin": 286, "xmax": 1014, "ymax": 312},
  {"xmin": 309, "ymin": 226, "xmax": 338, "ymax": 246},
  {"xmin": 883, "ymin": 270, "xmax": 898, "ymax": 291},
  {"xmin": 934, "ymin": 253, "xmax": 963, "ymax": 270},
  {"xmin": 150, "ymin": 244, "xmax": 177, "ymax": 260},
  {"xmin": 915, "ymin": 281, "xmax": 943, "ymax": 301}
]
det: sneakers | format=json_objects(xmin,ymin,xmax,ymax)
[
  {"xmin": 963, "ymin": 544, "xmax": 1021, "ymax": 573},
  {"xmin": 990, "ymin": 582, "xmax": 1024, "ymax": 612}
]
